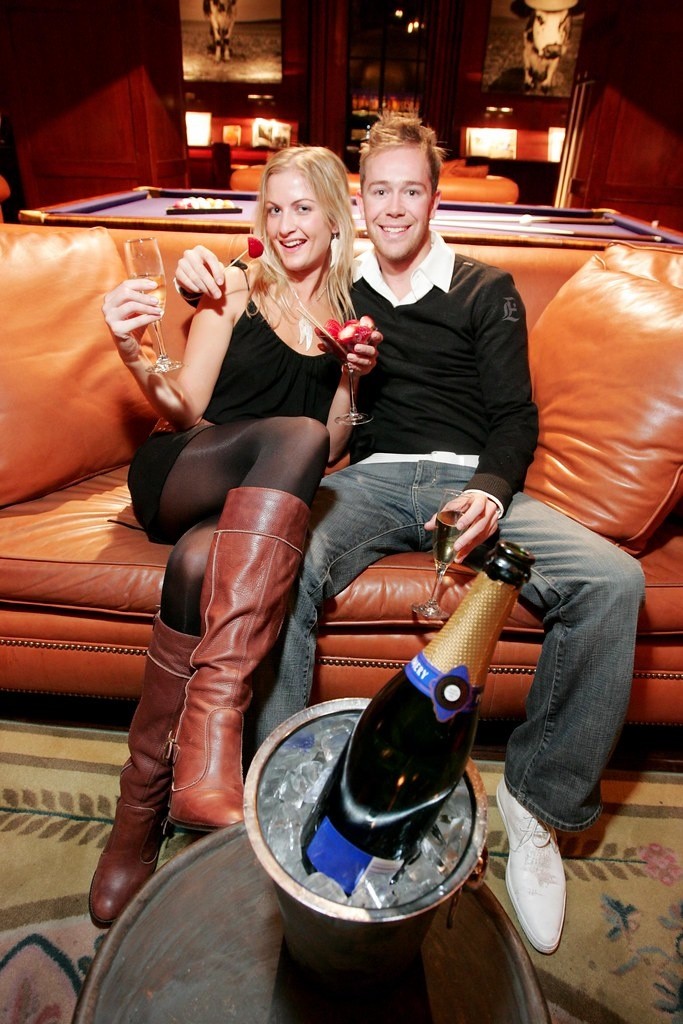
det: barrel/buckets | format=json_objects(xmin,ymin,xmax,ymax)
[{"xmin": 240, "ymin": 696, "xmax": 490, "ymax": 989}]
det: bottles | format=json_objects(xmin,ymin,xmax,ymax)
[{"xmin": 299, "ymin": 540, "xmax": 536, "ymax": 897}]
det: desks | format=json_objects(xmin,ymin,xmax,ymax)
[
  {"xmin": 15, "ymin": 183, "xmax": 683, "ymax": 244},
  {"xmin": 69, "ymin": 819, "xmax": 553, "ymax": 1024}
]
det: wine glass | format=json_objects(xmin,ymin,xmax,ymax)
[
  {"xmin": 410, "ymin": 489, "xmax": 475, "ymax": 620},
  {"xmin": 315, "ymin": 325, "xmax": 375, "ymax": 426},
  {"xmin": 123, "ymin": 236, "xmax": 186, "ymax": 375}
]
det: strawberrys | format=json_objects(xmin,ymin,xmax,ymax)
[
  {"xmin": 248, "ymin": 237, "xmax": 264, "ymax": 259},
  {"xmin": 314, "ymin": 316, "xmax": 374, "ymax": 363}
]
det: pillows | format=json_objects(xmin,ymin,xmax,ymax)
[{"xmin": 511, "ymin": 239, "xmax": 683, "ymax": 555}]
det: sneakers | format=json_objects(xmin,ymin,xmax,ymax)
[{"xmin": 495, "ymin": 778, "xmax": 567, "ymax": 955}]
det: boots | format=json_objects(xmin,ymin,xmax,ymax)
[
  {"xmin": 88, "ymin": 612, "xmax": 206, "ymax": 924},
  {"xmin": 159, "ymin": 486, "xmax": 314, "ymax": 830}
]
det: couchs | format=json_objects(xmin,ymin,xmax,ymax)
[{"xmin": 0, "ymin": 221, "xmax": 683, "ymax": 728}]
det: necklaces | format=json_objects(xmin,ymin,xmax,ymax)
[{"xmin": 290, "ymin": 286, "xmax": 328, "ymax": 350}]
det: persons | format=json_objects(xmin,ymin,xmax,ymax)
[
  {"xmin": 89, "ymin": 148, "xmax": 383, "ymax": 926},
  {"xmin": 174, "ymin": 113, "xmax": 645, "ymax": 954}
]
